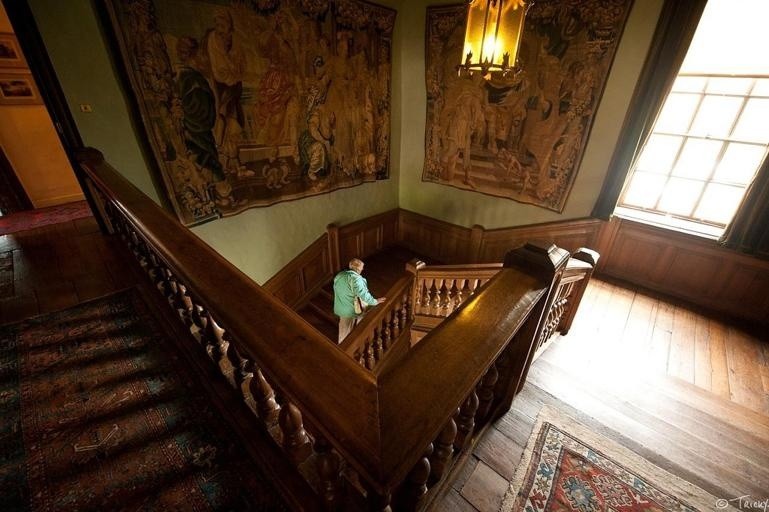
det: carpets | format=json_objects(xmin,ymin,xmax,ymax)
[
  {"xmin": 0, "ymin": 286, "xmax": 304, "ymax": 511},
  {"xmin": 0, "ymin": 197, "xmax": 95, "ymax": 237},
  {"xmin": 500, "ymin": 408, "xmax": 762, "ymax": 512}
]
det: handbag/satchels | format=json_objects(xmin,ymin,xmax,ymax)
[{"xmin": 354, "ymin": 296, "xmax": 368, "ymax": 316}]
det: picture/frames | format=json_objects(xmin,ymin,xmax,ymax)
[{"xmin": 0, "ymin": 32, "xmax": 42, "ymax": 106}]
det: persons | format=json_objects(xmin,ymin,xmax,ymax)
[
  {"xmin": 459, "ymin": 70, "xmax": 499, "ymax": 154},
  {"xmin": 331, "ymin": 257, "xmax": 387, "ymax": 344}
]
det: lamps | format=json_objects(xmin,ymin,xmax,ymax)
[{"xmin": 454, "ymin": 1, "xmax": 533, "ymax": 82}]
